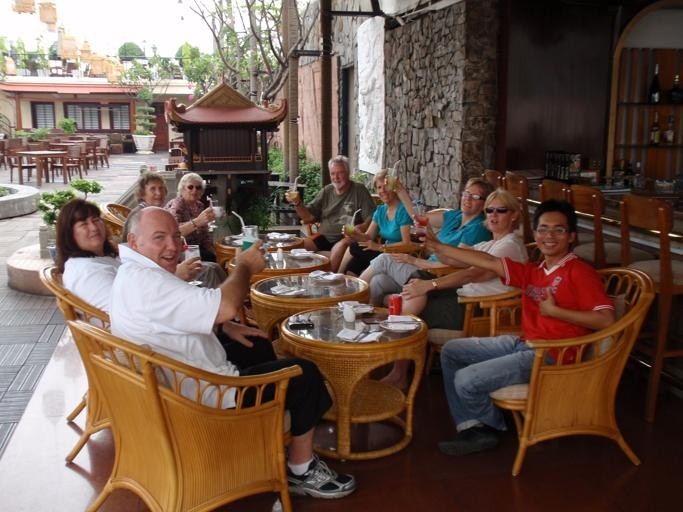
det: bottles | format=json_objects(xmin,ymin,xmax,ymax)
[
  {"xmin": 623, "ymin": 160, "xmax": 642, "ymax": 188},
  {"xmin": 650, "ymin": 111, "xmax": 661, "ymax": 144},
  {"xmin": 668, "ymin": 74, "xmax": 682, "ymax": 103},
  {"xmin": 649, "ymin": 63, "xmax": 661, "ymax": 103},
  {"xmin": 390, "ymin": 294, "xmax": 402, "ymax": 315},
  {"xmin": 662, "ymin": 115, "xmax": 675, "ymax": 143}
]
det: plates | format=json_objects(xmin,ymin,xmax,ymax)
[
  {"xmin": 288, "ymin": 252, "xmax": 314, "ymax": 259},
  {"xmin": 381, "ymin": 319, "xmax": 418, "ymax": 333},
  {"xmin": 338, "ymin": 303, "xmax": 375, "ymax": 315},
  {"xmin": 309, "ymin": 271, "xmax": 344, "ymax": 282}
]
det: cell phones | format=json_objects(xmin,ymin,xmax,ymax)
[{"xmin": 287, "ymin": 320, "xmax": 314, "ymax": 330}]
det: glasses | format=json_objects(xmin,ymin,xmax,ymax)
[
  {"xmin": 537, "ymin": 226, "xmax": 569, "ymax": 234},
  {"xmin": 462, "ymin": 190, "xmax": 485, "ymax": 201},
  {"xmin": 187, "ymin": 185, "xmax": 203, "ymax": 190},
  {"xmin": 485, "ymin": 207, "xmax": 508, "ymax": 213}
]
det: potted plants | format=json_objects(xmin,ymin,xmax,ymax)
[
  {"xmin": 182, "ymin": 41, "xmax": 192, "ymax": 80},
  {"xmin": 15, "ymin": 36, "xmax": 32, "ymax": 76},
  {"xmin": 35, "ymin": 48, "xmax": 49, "ymax": 75},
  {"xmin": 117, "ymin": 44, "xmax": 145, "ymax": 81},
  {"xmin": 35, "ymin": 189, "xmax": 77, "ymax": 257},
  {"xmin": 131, "ymin": 87, "xmax": 157, "ymax": 154}
]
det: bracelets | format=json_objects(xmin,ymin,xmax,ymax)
[{"xmin": 189, "ymin": 218, "xmax": 200, "ymax": 235}]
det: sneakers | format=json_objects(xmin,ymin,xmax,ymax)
[
  {"xmin": 286, "ymin": 453, "xmax": 356, "ymax": 499},
  {"xmin": 437, "ymin": 425, "xmax": 499, "ymax": 456}
]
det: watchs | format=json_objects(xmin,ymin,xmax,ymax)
[
  {"xmin": 217, "ymin": 322, "xmax": 225, "ymax": 335},
  {"xmin": 432, "ymin": 279, "xmax": 439, "ymax": 290}
]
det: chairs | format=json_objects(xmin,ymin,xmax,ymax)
[
  {"xmin": 480, "ymin": 269, "xmax": 654, "ymax": 476},
  {"xmin": 617, "ymin": 197, "xmax": 683, "ymax": 412},
  {"xmin": 428, "ymin": 242, "xmax": 573, "ymax": 378},
  {"xmin": 83, "ymin": 141, "xmax": 98, "ymax": 170},
  {"xmin": 344, "ymin": 207, "xmax": 454, "ymax": 281},
  {"xmin": 100, "ymin": 200, "xmax": 135, "ymax": 229},
  {"xmin": 66, "ymin": 320, "xmax": 303, "ymax": 511},
  {"xmin": 539, "ymin": 179, "xmax": 568, "ymax": 204},
  {"xmin": 93, "ymin": 140, "xmax": 111, "ymax": 171},
  {"xmin": 8, "ymin": 138, "xmax": 38, "ymax": 184},
  {"xmin": 502, "ymin": 171, "xmax": 536, "ymax": 252},
  {"xmin": 7, "ymin": 137, "xmax": 27, "ymax": 168},
  {"xmin": 51, "ymin": 146, "xmax": 84, "ymax": 182},
  {"xmin": 41, "ymin": 264, "xmax": 112, "ymax": 464},
  {"xmin": 97, "ymin": 218, "xmax": 132, "ymax": 255},
  {"xmin": 567, "ymin": 185, "xmax": 656, "ymax": 271}
]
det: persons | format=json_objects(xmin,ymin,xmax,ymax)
[
  {"xmin": 56, "ymin": 199, "xmax": 203, "ymax": 333},
  {"xmin": 123, "ymin": 173, "xmax": 227, "ymax": 289},
  {"xmin": 379, "ymin": 190, "xmax": 529, "ymax": 391},
  {"xmin": 109, "ymin": 206, "xmax": 356, "ymax": 500},
  {"xmin": 285, "ymin": 156, "xmax": 376, "ymax": 272},
  {"xmin": 161, "ymin": 173, "xmax": 226, "ymax": 262},
  {"xmin": 358, "ymin": 175, "xmax": 495, "ymax": 306},
  {"xmin": 419, "ymin": 199, "xmax": 616, "ymax": 456},
  {"xmin": 337, "ymin": 168, "xmax": 414, "ymax": 276}
]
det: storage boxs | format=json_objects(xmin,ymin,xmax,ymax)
[{"xmin": 482, "ymin": 169, "xmax": 502, "ymax": 194}]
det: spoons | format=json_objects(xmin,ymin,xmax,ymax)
[
  {"xmin": 354, "ymin": 325, "xmax": 370, "ymax": 341},
  {"xmin": 356, "ymin": 326, "xmax": 384, "ymax": 343}
]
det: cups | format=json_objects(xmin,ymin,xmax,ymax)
[
  {"xmin": 185, "ymin": 245, "xmax": 200, "ymax": 271},
  {"xmin": 412, "ymin": 206, "xmax": 427, "ymax": 228},
  {"xmin": 287, "ymin": 190, "xmax": 298, "ymax": 203},
  {"xmin": 387, "ymin": 168, "xmax": 397, "ymax": 190},
  {"xmin": 242, "ymin": 225, "xmax": 258, "ymax": 253},
  {"xmin": 410, "ymin": 226, "xmax": 426, "ymax": 243},
  {"xmin": 210, "ymin": 201, "xmax": 224, "ymax": 217},
  {"xmin": 343, "ymin": 224, "xmax": 353, "ymax": 237}
]
